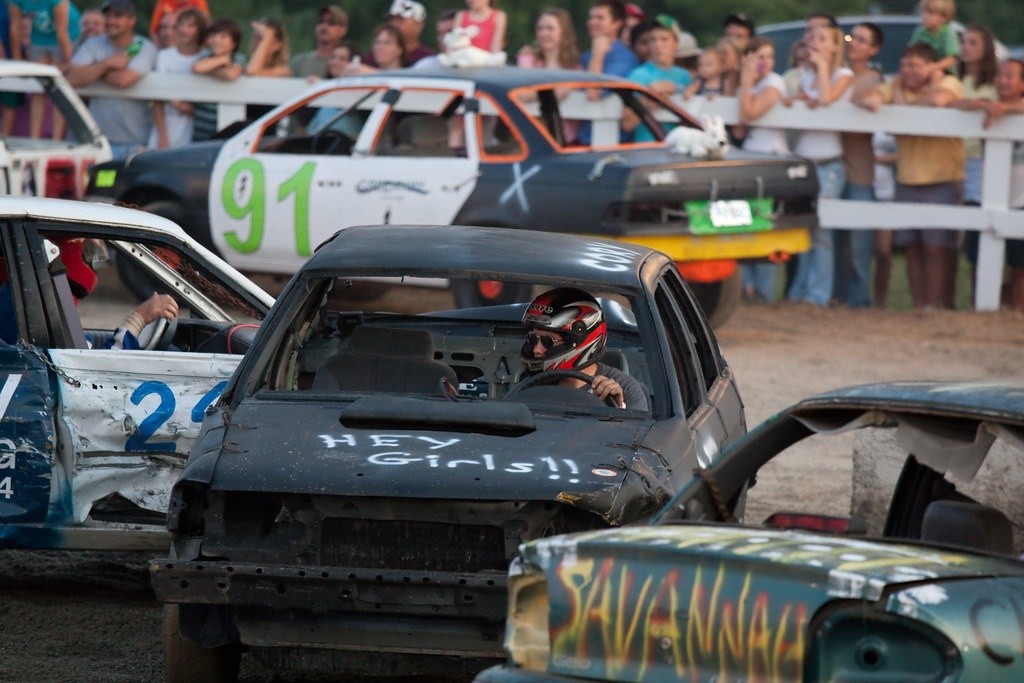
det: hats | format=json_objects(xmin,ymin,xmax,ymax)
[
  {"xmin": 381, "ymin": 0, "xmax": 427, "ymax": 24},
  {"xmin": 624, "ymin": 4, "xmax": 647, "ymax": 23},
  {"xmin": 656, "ymin": 11, "xmax": 679, "ymax": 39},
  {"xmin": 672, "ymin": 32, "xmax": 700, "ymax": 60},
  {"xmin": 320, "ymin": 5, "xmax": 349, "ymax": 24}
]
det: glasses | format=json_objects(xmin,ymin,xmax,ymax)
[
  {"xmin": 525, "ymin": 330, "xmax": 565, "ymax": 349},
  {"xmin": 316, "ymin": 17, "xmax": 337, "ymax": 25}
]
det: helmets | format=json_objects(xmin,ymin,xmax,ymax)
[
  {"xmin": 0, "ymin": 233, "xmax": 99, "ymax": 300},
  {"xmin": 520, "ymin": 287, "xmax": 607, "ymax": 376}
]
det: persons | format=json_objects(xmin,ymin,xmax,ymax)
[
  {"xmin": 50, "ymin": 238, "xmax": 178, "ymax": 350},
  {"xmin": 0, "ymin": 0, "xmax": 1024, "ymax": 308},
  {"xmin": 520, "ymin": 287, "xmax": 648, "ymax": 410}
]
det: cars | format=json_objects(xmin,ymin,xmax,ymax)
[
  {"xmin": 753, "ymin": 12, "xmax": 1024, "ymax": 72},
  {"xmin": 467, "ymin": 376, "xmax": 1023, "ymax": 683},
  {"xmin": 140, "ymin": 223, "xmax": 748, "ymax": 683},
  {"xmin": 0, "ymin": 194, "xmax": 295, "ymax": 559},
  {"xmin": 1, "ymin": 59, "xmax": 121, "ymax": 279}
]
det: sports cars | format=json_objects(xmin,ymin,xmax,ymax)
[{"xmin": 77, "ymin": 63, "xmax": 826, "ymax": 338}]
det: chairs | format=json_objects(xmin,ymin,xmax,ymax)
[
  {"xmin": 922, "ymin": 500, "xmax": 1014, "ymax": 556},
  {"xmin": 311, "ymin": 327, "xmax": 459, "ymax": 398},
  {"xmin": 382, "ymin": 113, "xmax": 458, "ymax": 158},
  {"xmin": 485, "ymin": 115, "xmax": 549, "ymax": 154}
]
